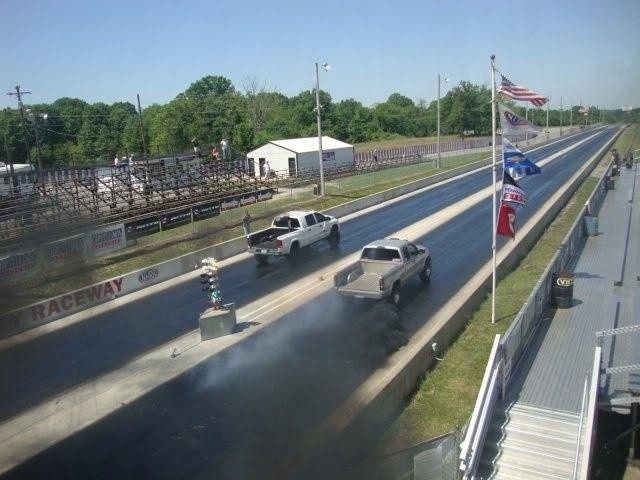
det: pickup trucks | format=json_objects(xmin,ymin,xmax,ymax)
[
  {"xmin": 247, "ymin": 207, "xmax": 342, "ymax": 265},
  {"xmin": 333, "ymin": 237, "xmax": 433, "ymax": 307}
]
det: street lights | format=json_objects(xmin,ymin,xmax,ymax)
[
  {"xmin": 313, "ymin": 61, "xmax": 333, "ymax": 199},
  {"xmin": 434, "ymin": 72, "xmax": 451, "ymax": 170}
]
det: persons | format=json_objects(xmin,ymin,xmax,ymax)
[
  {"xmin": 264, "ymin": 161, "xmax": 270, "ymax": 181},
  {"xmin": 242, "ymin": 209, "xmax": 251, "ymax": 235},
  {"xmin": 192, "ymin": 137, "xmax": 200, "ymax": 157},
  {"xmin": 221, "ymin": 137, "xmax": 229, "ymax": 159},
  {"xmin": 129, "ymin": 154, "xmax": 139, "ymax": 172},
  {"xmin": 213, "ymin": 147, "xmax": 222, "ymax": 160},
  {"xmin": 114, "ymin": 155, "xmax": 123, "ymax": 169}
]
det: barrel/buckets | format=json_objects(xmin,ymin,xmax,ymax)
[
  {"xmin": 606, "ymin": 180, "xmax": 614, "ymax": 190},
  {"xmin": 583, "ymin": 215, "xmax": 599, "ymax": 237},
  {"xmin": 550, "ymin": 272, "xmax": 574, "ymax": 309}
]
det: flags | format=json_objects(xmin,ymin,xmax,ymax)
[{"xmin": 496, "ymin": 76, "xmax": 550, "ymax": 240}]
